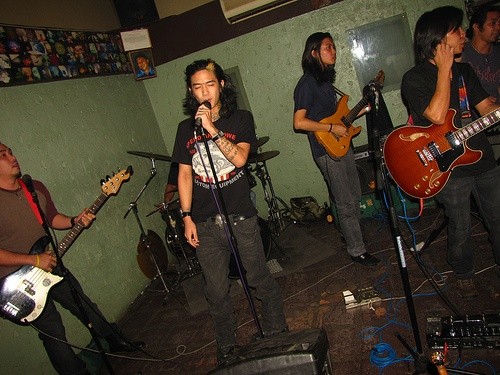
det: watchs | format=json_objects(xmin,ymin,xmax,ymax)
[
  {"xmin": 180, "ymin": 211, "xmax": 191, "ymax": 218},
  {"xmin": 212, "ymin": 130, "xmax": 224, "ymax": 141}
]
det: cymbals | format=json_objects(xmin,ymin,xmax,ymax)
[
  {"xmin": 253, "ymin": 135, "xmax": 270, "ymax": 146},
  {"xmin": 245, "ymin": 150, "xmax": 281, "ymax": 163},
  {"xmin": 125, "ymin": 150, "xmax": 181, "ymax": 163}
]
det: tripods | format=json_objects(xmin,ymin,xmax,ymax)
[
  {"xmin": 119, "ymin": 157, "xmax": 204, "ymax": 313},
  {"xmin": 256, "ymin": 148, "xmax": 309, "ymax": 240}
]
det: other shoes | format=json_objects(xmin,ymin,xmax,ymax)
[
  {"xmin": 217, "ymin": 345, "xmax": 236, "ymax": 365},
  {"xmin": 456, "ymin": 279, "xmax": 478, "ymax": 298}
]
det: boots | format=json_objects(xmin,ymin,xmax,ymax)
[{"xmin": 105, "ymin": 331, "xmax": 145, "ymax": 355}]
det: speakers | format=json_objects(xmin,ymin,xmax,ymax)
[
  {"xmin": 371, "ymin": 96, "xmax": 485, "ymax": 375},
  {"xmin": 204, "ymin": 327, "xmax": 334, "ymax": 374}
]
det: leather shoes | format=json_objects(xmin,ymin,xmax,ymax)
[{"xmin": 352, "ymin": 253, "xmax": 380, "ymax": 267}]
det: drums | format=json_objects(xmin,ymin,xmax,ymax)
[
  {"xmin": 164, "ymin": 219, "xmax": 198, "ymax": 259},
  {"xmin": 226, "ymin": 216, "xmax": 272, "ymax": 281}
]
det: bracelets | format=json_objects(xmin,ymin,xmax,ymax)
[
  {"xmin": 71, "ymin": 217, "xmax": 75, "ymax": 227},
  {"xmin": 365, "ymin": 106, "xmax": 370, "ymax": 112},
  {"xmin": 36, "ymin": 255, "xmax": 40, "ymax": 267},
  {"xmin": 329, "ymin": 123, "xmax": 332, "ymax": 132}
]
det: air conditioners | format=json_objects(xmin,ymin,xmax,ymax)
[{"xmin": 219, "ymin": 0, "xmax": 298, "ymax": 25}]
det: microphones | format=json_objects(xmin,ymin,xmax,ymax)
[
  {"xmin": 195, "ymin": 100, "xmax": 212, "ymax": 127},
  {"xmin": 368, "ymin": 79, "xmax": 376, "ymax": 91},
  {"xmin": 21, "ymin": 173, "xmax": 37, "ymax": 199}
]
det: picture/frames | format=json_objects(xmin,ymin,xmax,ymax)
[{"xmin": 129, "ymin": 49, "xmax": 157, "ymax": 81}]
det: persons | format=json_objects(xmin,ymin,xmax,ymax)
[
  {"xmin": 0, "ymin": 143, "xmax": 148, "ymax": 375},
  {"xmin": 164, "ymin": 162, "xmax": 178, "ymax": 204},
  {"xmin": 293, "ymin": 31, "xmax": 381, "ymax": 266},
  {"xmin": 400, "ymin": 6, "xmax": 500, "ymax": 301},
  {"xmin": 135, "ymin": 55, "xmax": 155, "ymax": 78},
  {"xmin": 172, "ymin": 59, "xmax": 287, "ymax": 349},
  {"xmin": 459, "ymin": 4, "xmax": 500, "ymax": 107}
]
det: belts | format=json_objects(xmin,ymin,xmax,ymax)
[{"xmin": 202, "ymin": 214, "xmax": 248, "ymax": 223}]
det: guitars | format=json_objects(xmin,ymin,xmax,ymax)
[
  {"xmin": 314, "ymin": 69, "xmax": 387, "ymax": 159},
  {"xmin": 382, "ymin": 106, "xmax": 500, "ymax": 200},
  {"xmin": 0, "ymin": 165, "xmax": 135, "ymax": 325}
]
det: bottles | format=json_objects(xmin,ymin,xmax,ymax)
[{"xmin": 324, "ymin": 202, "xmax": 334, "ymax": 224}]
